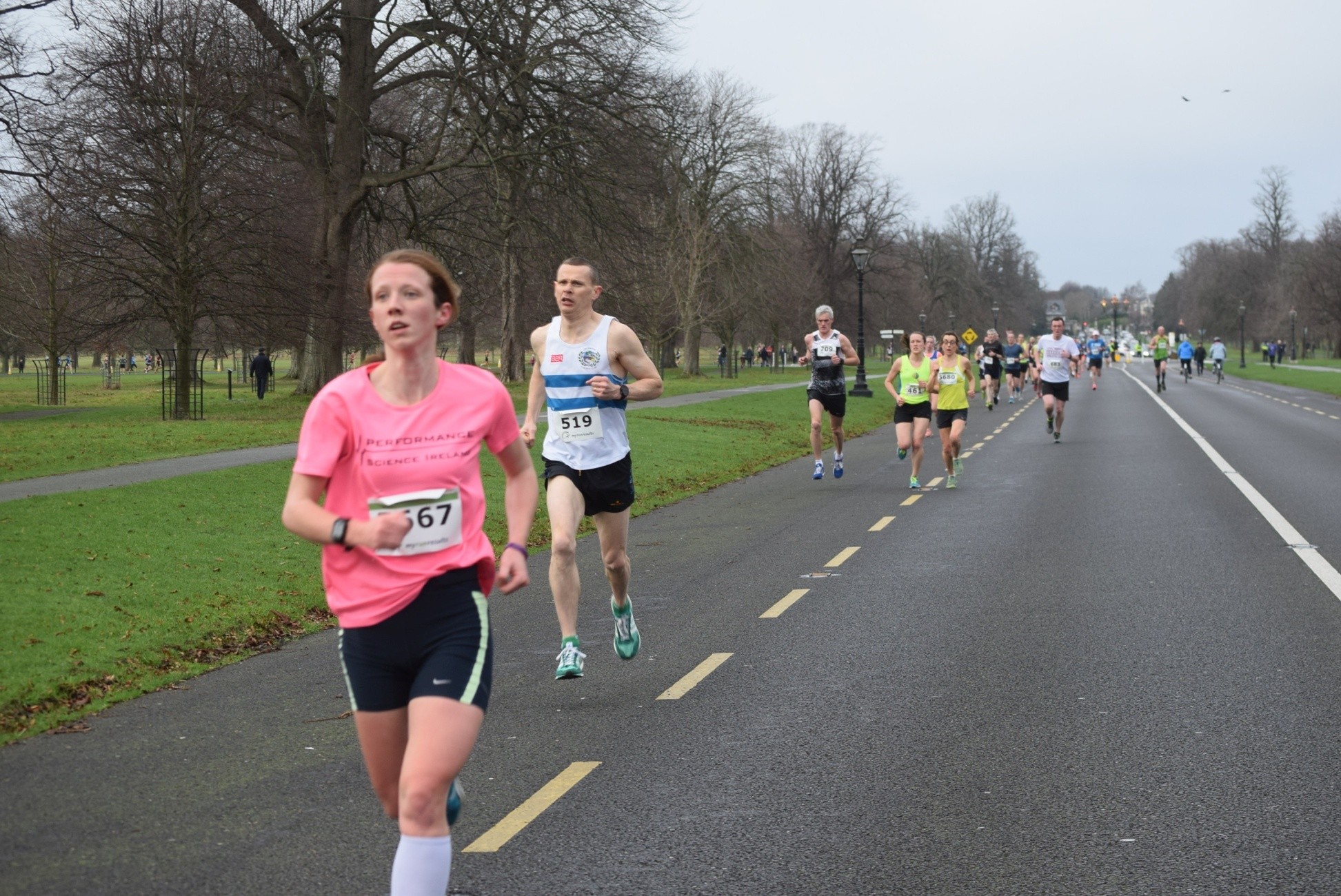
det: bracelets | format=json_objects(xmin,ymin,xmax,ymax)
[
  {"xmin": 504, "ymin": 543, "xmax": 530, "ymax": 561},
  {"xmin": 1067, "ymin": 354, "xmax": 1072, "ymax": 359}
]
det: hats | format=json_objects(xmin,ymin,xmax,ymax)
[{"xmin": 1213, "ymin": 336, "xmax": 1222, "ymax": 341}]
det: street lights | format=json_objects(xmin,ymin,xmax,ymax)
[
  {"xmin": 1238, "ymin": 300, "xmax": 1247, "ymax": 368},
  {"xmin": 1289, "ymin": 306, "xmax": 1297, "ymax": 359},
  {"xmin": 1101, "ymin": 294, "xmax": 1130, "ymax": 344}
]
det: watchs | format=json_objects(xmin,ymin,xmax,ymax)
[
  {"xmin": 331, "ymin": 517, "xmax": 355, "ymax": 552},
  {"xmin": 840, "ymin": 358, "xmax": 844, "ymax": 365},
  {"xmin": 618, "ymin": 384, "xmax": 629, "ymax": 401}
]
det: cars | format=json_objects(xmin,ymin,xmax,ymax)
[
  {"xmin": 1087, "ymin": 327, "xmax": 1112, "ymax": 337},
  {"xmin": 1119, "ymin": 330, "xmax": 1149, "ymax": 357}
]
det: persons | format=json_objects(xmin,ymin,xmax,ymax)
[
  {"xmin": 1268, "ymin": 341, "xmax": 1277, "ymax": 367},
  {"xmin": 1177, "ymin": 338, "xmax": 1195, "ymax": 379},
  {"xmin": 278, "ymin": 246, "xmax": 540, "ymax": 896},
  {"xmin": 1147, "ymin": 326, "xmax": 1170, "ymax": 393},
  {"xmin": 17, "ymin": 354, "xmax": 163, "ymax": 375},
  {"xmin": 925, "ymin": 330, "xmax": 976, "ymax": 489},
  {"xmin": 519, "ymin": 256, "xmax": 664, "ymax": 681},
  {"xmin": 936, "ymin": 328, "xmax": 1143, "ymax": 412},
  {"xmin": 1033, "ymin": 317, "xmax": 1080, "ymax": 444},
  {"xmin": 922, "ymin": 335, "xmax": 943, "ymax": 437},
  {"xmin": 884, "ymin": 332, "xmax": 934, "ymax": 488},
  {"xmin": 1194, "ymin": 342, "xmax": 1207, "ymax": 377},
  {"xmin": 1209, "ymin": 337, "xmax": 1227, "ymax": 380},
  {"xmin": 675, "ymin": 342, "xmax": 799, "ymax": 369},
  {"xmin": 1261, "ymin": 340, "xmax": 1269, "ymax": 362},
  {"xmin": 1275, "ymin": 339, "xmax": 1285, "ymax": 364},
  {"xmin": 249, "ymin": 346, "xmax": 273, "ymax": 400},
  {"xmin": 798, "ymin": 304, "xmax": 860, "ymax": 480},
  {"xmin": 480, "ymin": 349, "xmax": 536, "ymax": 377},
  {"xmin": 347, "ymin": 352, "xmax": 356, "ymax": 370},
  {"xmin": 885, "ymin": 342, "xmax": 894, "ymax": 362}
]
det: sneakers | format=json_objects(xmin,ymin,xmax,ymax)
[
  {"xmin": 953, "ymin": 456, "xmax": 964, "ymax": 475},
  {"xmin": 909, "ymin": 474, "xmax": 921, "ymax": 487},
  {"xmin": 946, "ymin": 475, "xmax": 958, "ymax": 488},
  {"xmin": 832, "ymin": 451, "xmax": 844, "ymax": 478},
  {"xmin": 1054, "ymin": 431, "xmax": 1060, "ymax": 443},
  {"xmin": 1045, "ymin": 415, "xmax": 1055, "ymax": 434},
  {"xmin": 611, "ymin": 592, "xmax": 642, "ymax": 660},
  {"xmin": 554, "ymin": 636, "xmax": 588, "ymax": 680},
  {"xmin": 812, "ymin": 463, "xmax": 825, "ymax": 479},
  {"xmin": 896, "ymin": 447, "xmax": 907, "ymax": 460}
]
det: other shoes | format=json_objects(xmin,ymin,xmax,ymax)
[
  {"xmin": 1156, "ymin": 383, "xmax": 1162, "ymax": 393},
  {"xmin": 1092, "ymin": 383, "xmax": 1098, "ymax": 389},
  {"xmin": 1006, "ymin": 397, "xmax": 1015, "ymax": 403},
  {"xmin": 1161, "ymin": 383, "xmax": 1166, "ymax": 390},
  {"xmin": 925, "ymin": 427, "xmax": 933, "ymax": 437},
  {"xmin": 993, "ymin": 395, "xmax": 998, "ymax": 406},
  {"xmin": 981, "ymin": 390, "xmax": 987, "ymax": 399},
  {"xmin": 985, "ymin": 401, "xmax": 993, "ymax": 409}
]
